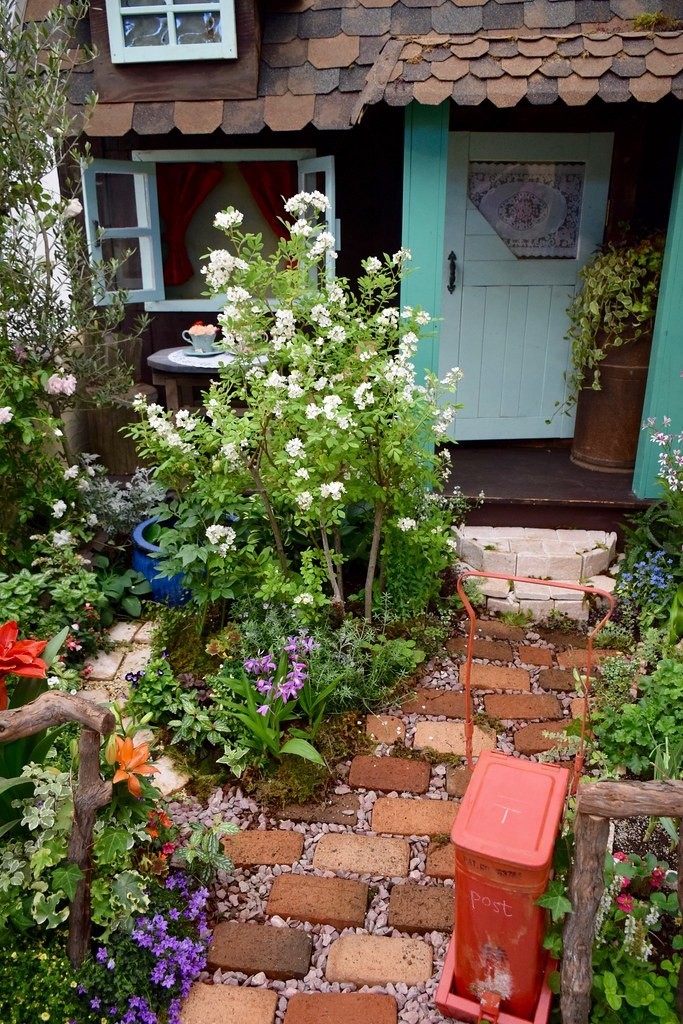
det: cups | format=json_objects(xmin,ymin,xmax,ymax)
[
  {"xmin": 182, "ymin": 330, "xmax": 216, "ymax": 352},
  {"xmin": 243, "ymin": 326, "xmax": 268, "ymax": 343}
]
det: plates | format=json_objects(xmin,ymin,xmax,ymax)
[{"xmin": 184, "ymin": 348, "xmax": 224, "ymax": 355}]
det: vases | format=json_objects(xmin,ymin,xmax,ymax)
[{"xmin": 131, "ymin": 508, "xmax": 239, "ymax": 608}]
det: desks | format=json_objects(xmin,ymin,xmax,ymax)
[{"xmin": 146, "ymin": 346, "xmax": 287, "ymax": 446}]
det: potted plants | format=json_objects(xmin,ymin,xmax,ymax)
[{"xmin": 542, "ymin": 241, "xmax": 663, "ymax": 474}]
